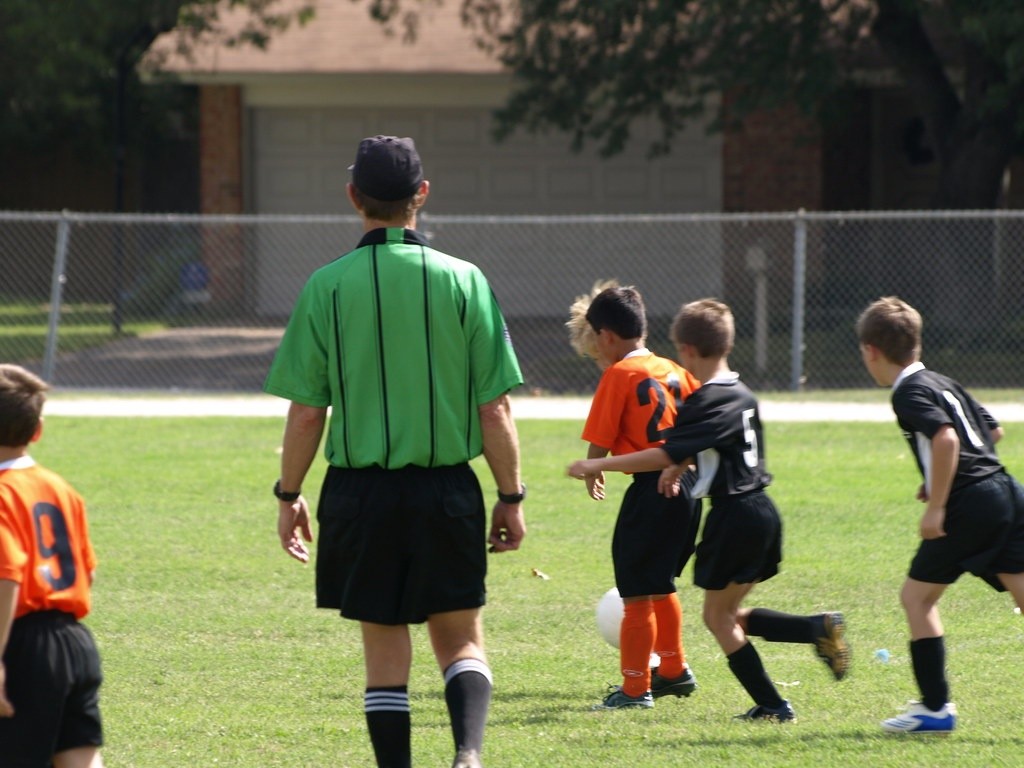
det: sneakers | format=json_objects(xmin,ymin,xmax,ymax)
[
  {"xmin": 650, "ymin": 663, "xmax": 695, "ymax": 698},
  {"xmin": 738, "ymin": 700, "xmax": 796, "ymax": 723},
  {"xmin": 882, "ymin": 700, "xmax": 956, "ymax": 733},
  {"xmin": 594, "ymin": 681, "xmax": 655, "ymax": 708},
  {"xmin": 817, "ymin": 611, "xmax": 848, "ymax": 679}
]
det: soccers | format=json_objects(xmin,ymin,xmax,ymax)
[{"xmin": 595, "ymin": 586, "xmax": 626, "ymax": 649}]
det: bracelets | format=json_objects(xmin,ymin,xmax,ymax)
[
  {"xmin": 274, "ymin": 479, "xmax": 301, "ymax": 501},
  {"xmin": 498, "ymin": 484, "xmax": 525, "ymax": 503}
]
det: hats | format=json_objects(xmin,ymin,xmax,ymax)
[{"xmin": 347, "ymin": 134, "xmax": 423, "ymax": 200}]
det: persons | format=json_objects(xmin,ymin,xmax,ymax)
[
  {"xmin": 571, "ymin": 282, "xmax": 701, "ymax": 712},
  {"xmin": 568, "ymin": 300, "xmax": 851, "ymax": 725},
  {"xmin": 0, "ymin": 364, "xmax": 103, "ymax": 768},
  {"xmin": 264, "ymin": 136, "xmax": 526, "ymax": 768},
  {"xmin": 857, "ymin": 296, "xmax": 1024, "ymax": 733}
]
